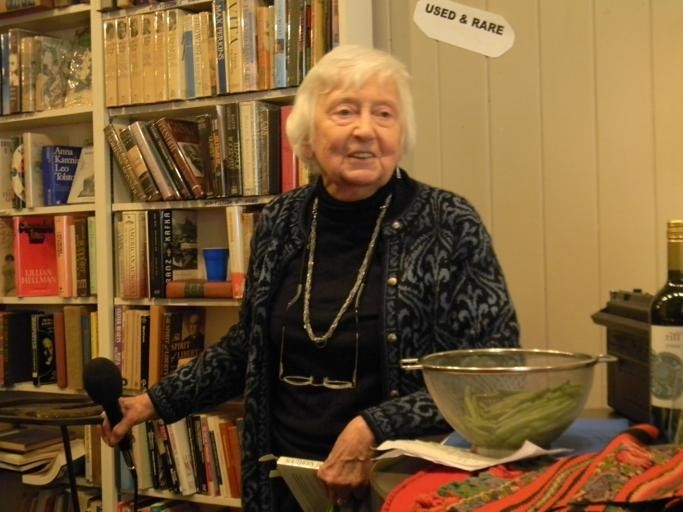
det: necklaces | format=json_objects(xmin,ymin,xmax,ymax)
[{"xmin": 303, "ymin": 196, "xmax": 391, "ymax": 344}]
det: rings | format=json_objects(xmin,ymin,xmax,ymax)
[{"xmin": 335, "ymin": 497, "xmax": 350, "ymax": 507}]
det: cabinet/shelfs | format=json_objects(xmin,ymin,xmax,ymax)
[{"xmin": 0, "ymin": 3, "xmax": 373, "ymax": 511}]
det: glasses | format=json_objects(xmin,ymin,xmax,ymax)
[{"xmin": 277, "ymin": 286, "xmax": 363, "ymax": 391}]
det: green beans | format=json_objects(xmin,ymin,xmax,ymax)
[{"xmin": 445, "ymin": 383, "xmax": 587, "ymax": 450}]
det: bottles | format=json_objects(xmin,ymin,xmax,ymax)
[{"xmin": 650, "ymin": 218, "xmax": 683, "ymax": 445}]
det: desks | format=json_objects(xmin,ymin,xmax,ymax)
[{"xmin": 362, "ymin": 408, "xmax": 683, "ymax": 512}]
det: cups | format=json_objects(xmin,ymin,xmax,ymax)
[{"xmin": 202, "ymin": 245, "xmax": 229, "ymax": 281}]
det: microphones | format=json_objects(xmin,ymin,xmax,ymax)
[{"xmin": 82, "ymin": 356, "xmax": 137, "ymax": 481}]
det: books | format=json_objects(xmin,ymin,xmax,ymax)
[
  {"xmin": 113, "ymin": 306, "xmax": 207, "ymax": 391},
  {"xmin": 276, "ymin": 457, "xmax": 338, "ymax": 512},
  {"xmin": 104, "ymin": 101, "xmax": 310, "ymax": 203},
  {"xmin": 113, "ymin": 206, "xmax": 261, "ymax": 300},
  {"xmin": 2, "ymin": 425, "xmax": 100, "ymax": 511},
  {"xmin": 2, "ymin": 0, "xmax": 91, "ymax": 115},
  {"xmin": 114, "ymin": 411, "xmax": 247, "ymax": 511},
  {"xmin": 103, "ymin": 0, "xmax": 339, "ymax": 107},
  {"xmin": 1, "ymin": 131, "xmax": 101, "ymax": 389}
]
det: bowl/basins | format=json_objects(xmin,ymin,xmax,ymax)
[{"xmin": 399, "ymin": 345, "xmax": 618, "ymax": 458}]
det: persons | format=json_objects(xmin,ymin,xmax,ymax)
[{"xmin": 100, "ymin": 44, "xmax": 522, "ymax": 511}]
